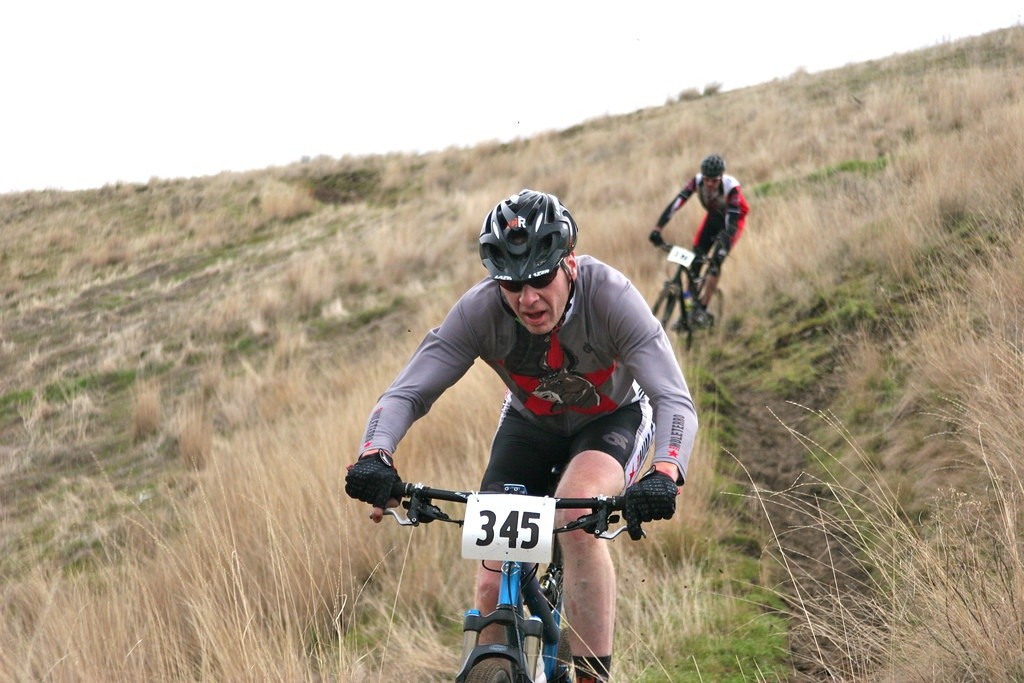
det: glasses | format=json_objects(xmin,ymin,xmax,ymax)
[
  {"xmin": 497, "ymin": 259, "xmax": 563, "ymax": 293},
  {"xmin": 702, "ymin": 178, "xmax": 723, "ymax": 183}
]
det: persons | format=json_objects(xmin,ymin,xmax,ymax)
[
  {"xmin": 345, "ymin": 189, "xmax": 698, "ymax": 683},
  {"xmin": 648, "ymin": 154, "xmax": 749, "ymax": 328}
]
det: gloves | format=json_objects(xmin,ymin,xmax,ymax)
[
  {"xmin": 623, "ymin": 466, "xmax": 678, "ymax": 541},
  {"xmin": 706, "ymin": 258, "xmax": 722, "ymax": 278},
  {"xmin": 648, "ymin": 231, "xmax": 664, "ymax": 247}
]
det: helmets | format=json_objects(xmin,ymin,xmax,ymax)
[
  {"xmin": 476, "ymin": 188, "xmax": 578, "ymax": 282},
  {"xmin": 701, "ymin": 154, "xmax": 725, "ymax": 176}
]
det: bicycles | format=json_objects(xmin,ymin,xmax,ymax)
[
  {"xmin": 369, "ymin": 481, "xmax": 650, "ymax": 683},
  {"xmin": 651, "ymin": 244, "xmax": 725, "ymax": 353}
]
